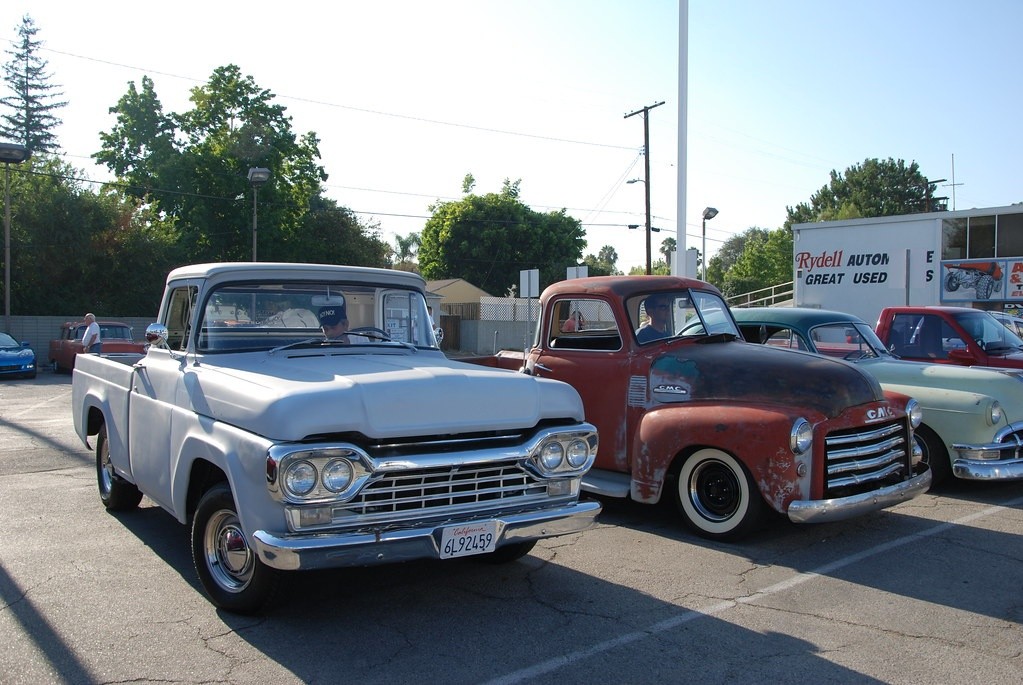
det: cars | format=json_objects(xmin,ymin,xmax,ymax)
[{"xmin": 675, "ymin": 307, "xmax": 1023, "ymax": 493}]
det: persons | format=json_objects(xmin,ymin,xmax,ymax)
[
  {"xmin": 318, "ymin": 306, "xmax": 370, "ymax": 346},
  {"xmin": 561, "ymin": 310, "xmax": 587, "ymax": 332},
  {"xmin": 82, "ymin": 312, "xmax": 102, "ymax": 353},
  {"xmin": 412, "ymin": 305, "xmax": 437, "ymax": 345},
  {"xmin": 637, "ymin": 294, "xmax": 673, "ymax": 343}
]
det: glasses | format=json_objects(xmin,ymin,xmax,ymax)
[{"xmin": 652, "ymin": 304, "xmax": 670, "ymax": 310}]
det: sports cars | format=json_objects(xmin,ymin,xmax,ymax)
[{"xmin": 0, "ymin": 331, "xmax": 37, "ymax": 379}]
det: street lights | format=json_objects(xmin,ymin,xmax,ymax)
[
  {"xmin": 247, "ymin": 167, "xmax": 268, "ymax": 322},
  {"xmin": 0, "ymin": 142, "xmax": 30, "ymax": 316},
  {"xmin": 701, "ymin": 207, "xmax": 719, "ymax": 281},
  {"xmin": 626, "ymin": 179, "xmax": 651, "ymax": 276}
]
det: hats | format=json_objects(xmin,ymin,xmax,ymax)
[{"xmin": 318, "ymin": 306, "xmax": 347, "ymax": 329}]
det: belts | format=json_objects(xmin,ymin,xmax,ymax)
[{"xmin": 90, "ymin": 343, "xmax": 100, "ymax": 348}]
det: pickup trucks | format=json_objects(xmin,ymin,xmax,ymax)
[
  {"xmin": 72, "ymin": 261, "xmax": 602, "ymax": 616},
  {"xmin": 764, "ymin": 306, "xmax": 1023, "ymax": 369},
  {"xmin": 445, "ymin": 276, "xmax": 932, "ymax": 542},
  {"xmin": 48, "ymin": 322, "xmax": 151, "ymax": 375}
]
